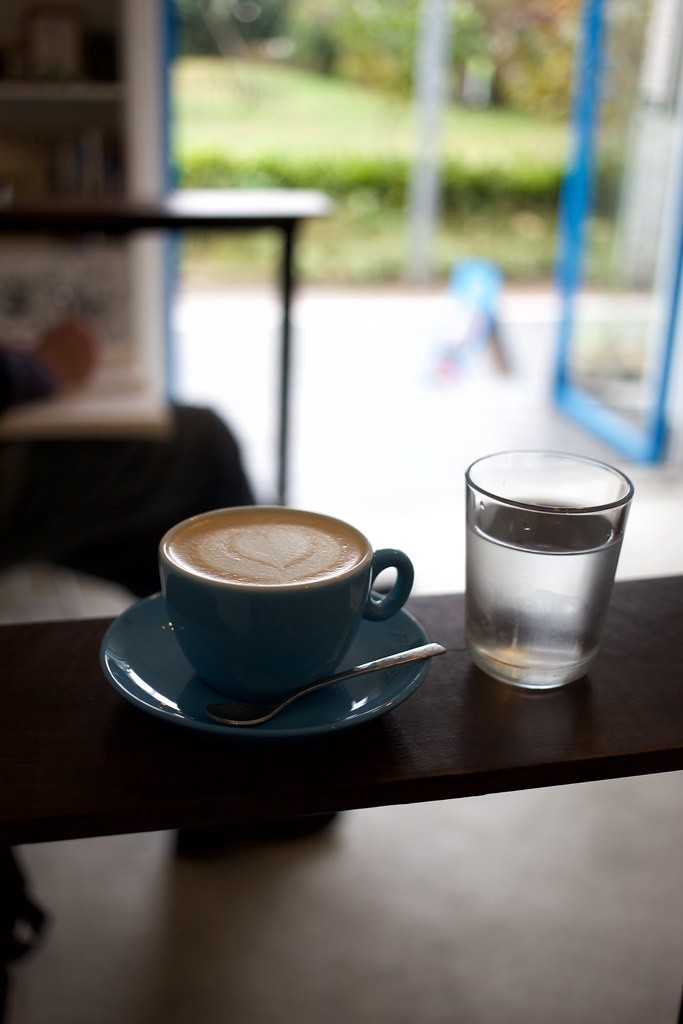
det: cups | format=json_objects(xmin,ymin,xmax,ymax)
[
  {"xmin": 466, "ymin": 451, "xmax": 633, "ymax": 690},
  {"xmin": 157, "ymin": 505, "xmax": 415, "ymax": 694}
]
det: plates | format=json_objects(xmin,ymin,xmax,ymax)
[{"xmin": 99, "ymin": 591, "xmax": 432, "ymax": 737}]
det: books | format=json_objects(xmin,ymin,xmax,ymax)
[{"xmin": 0, "ymin": 365, "xmax": 171, "ymax": 440}]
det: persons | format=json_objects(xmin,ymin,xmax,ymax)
[{"xmin": 0, "ymin": 314, "xmax": 259, "ymax": 596}]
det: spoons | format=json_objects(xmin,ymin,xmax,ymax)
[{"xmin": 206, "ymin": 640, "xmax": 447, "ymax": 723}]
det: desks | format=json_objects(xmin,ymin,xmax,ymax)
[{"xmin": 0, "ymin": 185, "xmax": 336, "ymax": 511}]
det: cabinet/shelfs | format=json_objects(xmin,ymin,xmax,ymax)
[{"xmin": 0, "ymin": 0, "xmax": 161, "ymax": 425}]
